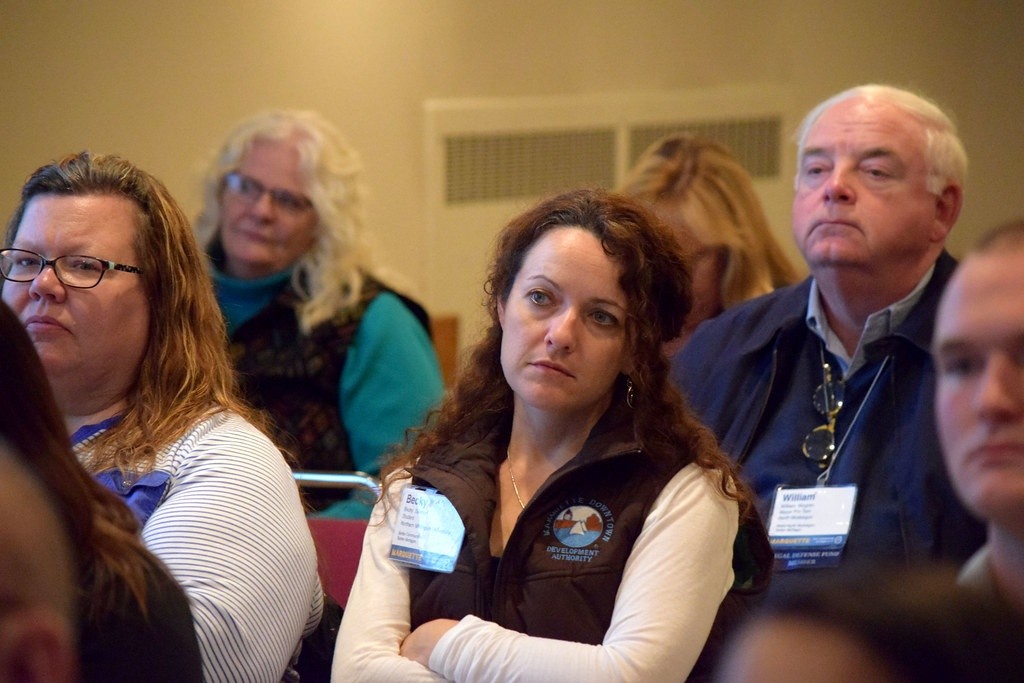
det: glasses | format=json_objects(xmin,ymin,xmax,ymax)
[
  {"xmin": 224, "ymin": 171, "xmax": 312, "ymax": 214},
  {"xmin": 0, "ymin": 249, "xmax": 148, "ymax": 288},
  {"xmin": 803, "ymin": 362, "xmax": 845, "ymax": 470}
]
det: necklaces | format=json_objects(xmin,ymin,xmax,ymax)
[{"xmin": 506, "ymin": 449, "xmax": 524, "ymax": 510}]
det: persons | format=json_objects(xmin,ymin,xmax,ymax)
[
  {"xmin": 333, "ymin": 188, "xmax": 774, "ymax": 683},
  {"xmin": 671, "ymin": 83, "xmax": 988, "ymax": 593},
  {"xmin": 928, "ymin": 221, "xmax": 1024, "ymax": 589},
  {"xmin": 616, "ymin": 131, "xmax": 798, "ymax": 356},
  {"xmin": 1, "ymin": 301, "xmax": 204, "ymax": 683},
  {"xmin": 188, "ymin": 104, "xmax": 447, "ymax": 520},
  {"xmin": 722, "ymin": 563, "xmax": 1024, "ymax": 683},
  {"xmin": 0, "ymin": 152, "xmax": 323, "ymax": 683}
]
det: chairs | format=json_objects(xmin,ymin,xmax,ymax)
[{"xmin": 294, "ymin": 471, "xmax": 382, "ymax": 609}]
